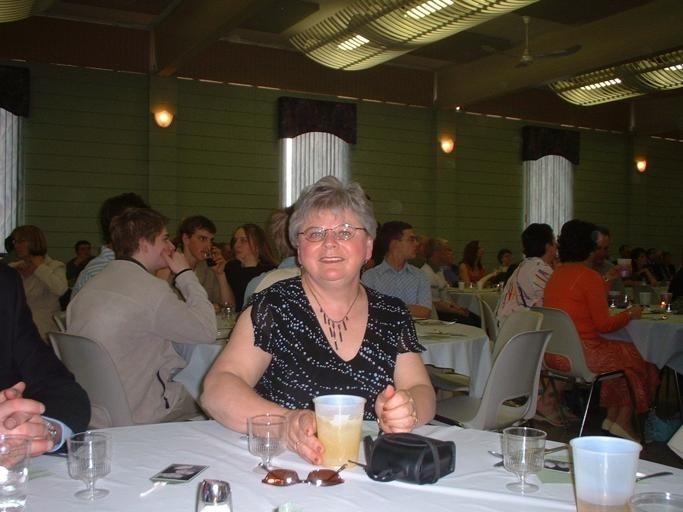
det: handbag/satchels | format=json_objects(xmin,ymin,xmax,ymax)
[
  {"xmin": 643, "ymin": 407, "xmax": 682, "ymax": 443},
  {"xmin": 367, "ymin": 432, "xmax": 456, "ymax": 485}
]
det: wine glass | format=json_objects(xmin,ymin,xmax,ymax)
[
  {"xmin": 64, "ymin": 430, "xmax": 113, "ymax": 500},
  {"xmin": 248, "ymin": 413, "xmax": 288, "ymax": 473},
  {"xmin": 0, "ymin": 431, "xmax": 32, "ymax": 512},
  {"xmin": 500, "ymin": 425, "xmax": 546, "ymax": 493}
]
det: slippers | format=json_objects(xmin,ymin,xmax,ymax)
[{"xmin": 533, "ymin": 403, "xmax": 580, "ymax": 428}]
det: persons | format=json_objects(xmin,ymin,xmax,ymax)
[
  {"xmin": 0, "ymin": 262, "xmax": 94, "ymax": 462},
  {"xmin": 198, "ymin": 175, "xmax": 443, "ymax": 468},
  {"xmin": 0, "ymin": 193, "xmax": 301, "ymax": 432},
  {"xmin": 361, "ymin": 194, "xmax": 683, "ymax": 457}
]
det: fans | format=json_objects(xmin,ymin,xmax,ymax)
[{"xmin": 483, "ymin": 16, "xmax": 580, "ymax": 72}]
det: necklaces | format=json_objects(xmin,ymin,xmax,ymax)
[{"xmin": 303, "ymin": 274, "xmax": 361, "ymax": 351}]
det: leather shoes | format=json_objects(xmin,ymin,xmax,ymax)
[{"xmin": 600, "ymin": 418, "xmax": 642, "ymax": 444}]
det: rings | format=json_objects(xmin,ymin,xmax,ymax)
[{"xmin": 291, "ymin": 440, "xmax": 305, "ymax": 450}]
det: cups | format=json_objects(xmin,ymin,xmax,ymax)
[
  {"xmin": 629, "ymin": 490, "xmax": 683, "ymax": 512},
  {"xmin": 313, "ymin": 394, "xmax": 365, "ymax": 468},
  {"xmin": 210, "ymin": 302, "xmax": 233, "ymax": 339},
  {"xmin": 608, "ymin": 285, "xmax": 675, "ymax": 319},
  {"xmin": 618, "ymin": 258, "xmax": 633, "ymax": 278},
  {"xmin": 199, "ymin": 479, "xmax": 233, "ymax": 512},
  {"xmin": 459, "ymin": 282, "xmax": 465, "ymax": 291},
  {"xmin": 203, "ymin": 246, "xmax": 219, "ymax": 267},
  {"xmin": 570, "ymin": 436, "xmax": 642, "ymax": 511}
]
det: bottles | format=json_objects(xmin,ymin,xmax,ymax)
[{"xmin": 637, "ymin": 272, "xmax": 653, "ymax": 308}]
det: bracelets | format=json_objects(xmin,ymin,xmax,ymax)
[{"xmin": 45, "ymin": 418, "xmax": 59, "ymax": 445}]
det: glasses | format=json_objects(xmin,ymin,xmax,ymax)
[
  {"xmin": 261, "ymin": 464, "xmax": 349, "ymax": 487},
  {"xmin": 297, "ymin": 223, "xmax": 368, "ymax": 243}
]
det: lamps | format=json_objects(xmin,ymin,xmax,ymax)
[
  {"xmin": 545, "ymin": 44, "xmax": 682, "ymax": 108},
  {"xmin": 285, "ymin": 1, "xmax": 542, "ymax": 73}
]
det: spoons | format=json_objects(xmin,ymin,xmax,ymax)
[{"xmin": 487, "ymin": 445, "xmax": 569, "ymax": 459}]
